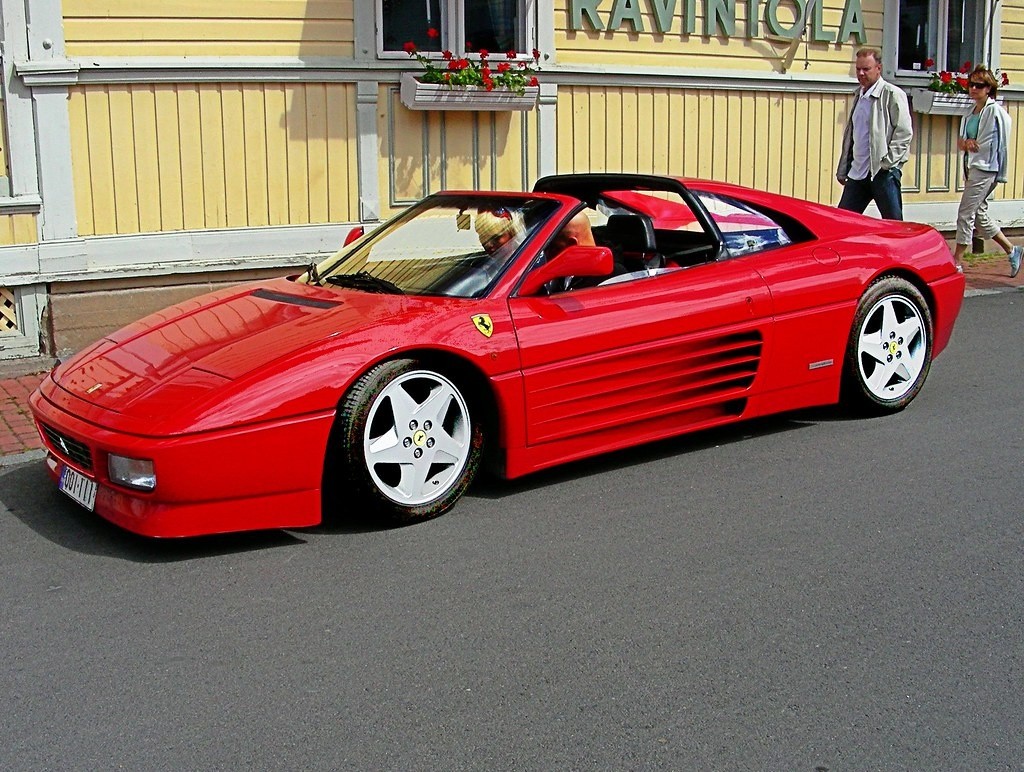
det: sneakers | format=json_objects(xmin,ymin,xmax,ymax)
[
  {"xmin": 955, "ymin": 266, "xmax": 963, "ymax": 273},
  {"xmin": 1009, "ymin": 246, "xmax": 1024, "ymax": 278}
]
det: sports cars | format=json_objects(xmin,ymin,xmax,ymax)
[{"xmin": 24, "ymin": 172, "xmax": 967, "ymax": 539}]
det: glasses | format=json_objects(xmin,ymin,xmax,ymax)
[
  {"xmin": 968, "ymin": 82, "xmax": 987, "ymax": 89},
  {"xmin": 477, "ymin": 204, "xmax": 512, "ymax": 222}
]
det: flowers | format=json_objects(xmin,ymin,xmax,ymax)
[
  {"xmin": 402, "ymin": 27, "xmax": 542, "ymax": 97},
  {"xmin": 923, "ymin": 58, "xmax": 1010, "ymax": 95}
]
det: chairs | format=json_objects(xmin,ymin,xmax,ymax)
[{"xmin": 607, "ymin": 212, "xmax": 661, "ymax": 275}]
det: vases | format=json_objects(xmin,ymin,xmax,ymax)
[
  {"xmin": 400, "ymin": 71, "xmax": 539, "ymax": 112},
  {"xmin": 910, "ymin": 88, "xmax": 1004, "ymax": 116}
]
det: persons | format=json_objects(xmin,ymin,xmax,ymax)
[
  {"xmin": 953, "ymin": 69, "xmax": 1024, "ymax": 278},
  {"xmin": 837, "ymin": 47, "xmax": 913, "ymax": 222},
  {"xmin": 475, "ymin": 207, "xmax": 628, "ymax": 295}
]
